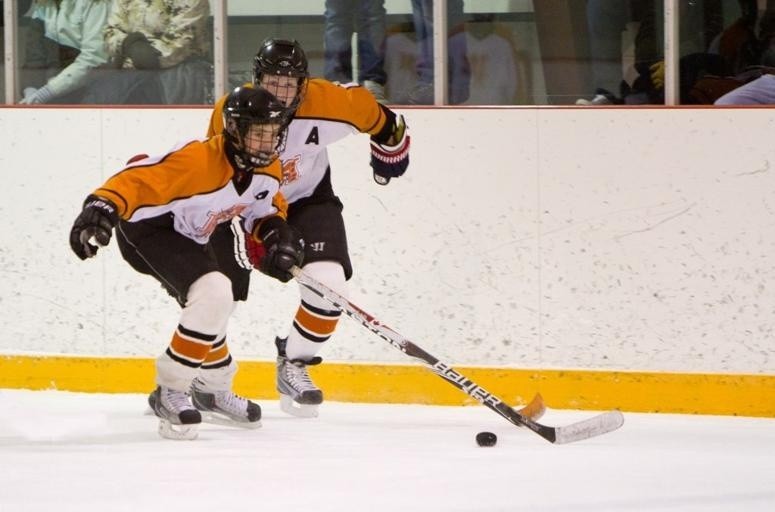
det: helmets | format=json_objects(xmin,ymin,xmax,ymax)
[
  {"xmin": 222, "ymin": 86, "xmax": 291, "ymax": 175},
  {"xmin": 252, "ymin": 37, "xmax": 309, "ymax": 118}
]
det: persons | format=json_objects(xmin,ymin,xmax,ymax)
[
  {"xmin": 574, "ymin": 0, "xmax": 775, "ymax": 106},
  {"xmin": 409, "ymin": 0, "xmax": 471, "ymax": 106},
  {"xmin": 69, "ymin": 85, "xmax": 309, "ymax": 427},
  {"xmin": 108, "ymin": 2, "xmax": 212, "ymax": 107},
  {"xmin": 190, "ymin": 39, "xmax": 410, "ymax": 414},
  {"xmin": 323, "ymin": 0, "xmax": 387, "ymax": 105},
  {"xmin": 17, "ymin": 1, "xmax": 112, "ymax": 105}
]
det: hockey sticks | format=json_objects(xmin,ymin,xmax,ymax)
[
  {"xmin": 291, "ymin": 267, "xmax": 545, "ymax": 427},
  {"xmin": 288, "ymin": 264, "xmax": 624, "ymax": 443}
]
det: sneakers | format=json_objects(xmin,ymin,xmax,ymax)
[
  {"xmin": 364, "ymin": 80, "xmax": 385, "ymax": 105},
  {"xmin": 408, "ymin": 84, "xmax": 471, "ymax": 105},
  {"xmin": 391, "ymin": 81, "xmax": 428, "ymax": 105},
  {"xmin": 187, "ymin": 378, "xmax": 261, "ymax": 423},
  {"xmin": 147, "ymin": 385, "xmax": 202, "ymax": 425},
  {"xmin": 274, "ymin": 335, "xmax": 324, "ymax": 407}
]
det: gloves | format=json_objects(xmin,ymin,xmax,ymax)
[
  {"xmin": 369, "ymin": 115, "xmax": 411, "ymax": 185},
  {"xmin": 259, "ymin": 219, "xmax": 305, "ymax": 283},
  {"xmin": 120, "ymin": 31, "xmax": 160, "ymax": 70},
  {"xmin": 69, "ymin": 194, "xmax": 120, "ymax": 261}
]
